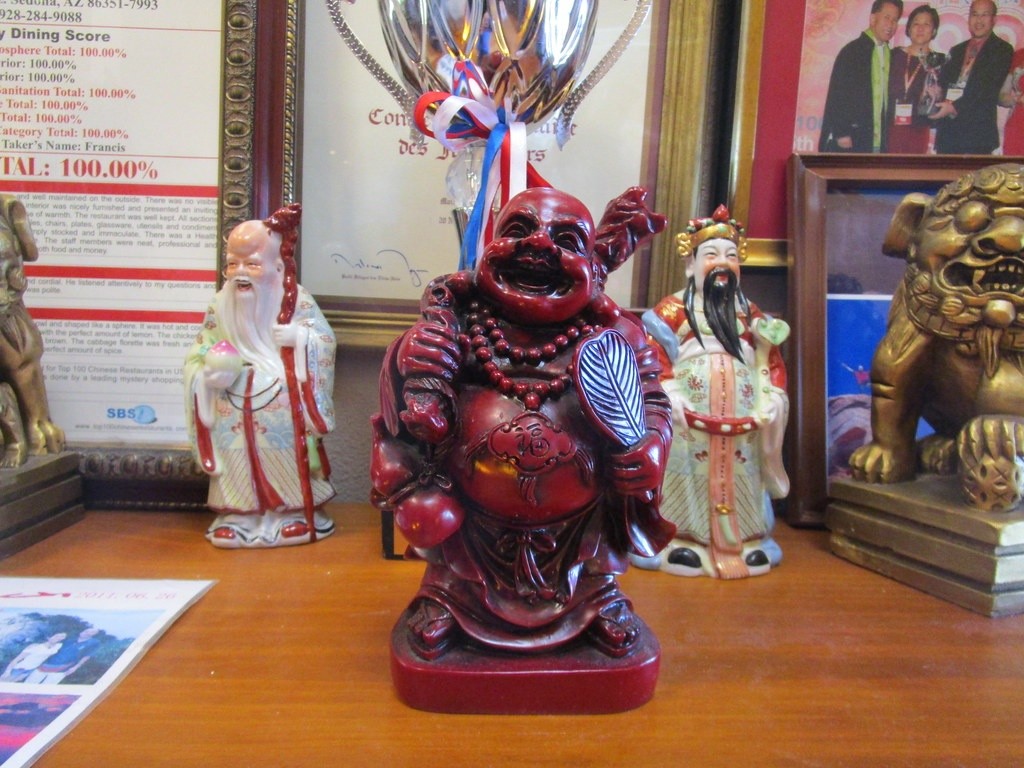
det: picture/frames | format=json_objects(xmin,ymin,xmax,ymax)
[
  {"xmin": 725, "ymin": 0, "xmax": 1024, "ymax": 272},
  {"xmin": 786, "ymin": 152, "xmax": 1024, "ymax": 527}
]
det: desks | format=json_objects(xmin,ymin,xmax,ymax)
[{"xmin": 0, "ymin": 501, "xmax": 1024, "ymax": 768}]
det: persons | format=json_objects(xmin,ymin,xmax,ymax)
[
  {"xmin": 817, "ymin": 1, "xmax": 1014, "ymax": 157},
  {"xmin": 175, "ymin": 205, "xmax": 346, "ymax": 553},
  {"xmin": 371, "ymin": 186, "xmax": 670, "ymax": 663},
  {"xmin": 638, "ymin": 205, "xmax": 796, "ymax": 578},
  {"xmin": 0, "ymin": 628, "xmax": 102, "ymax": 686}
]
